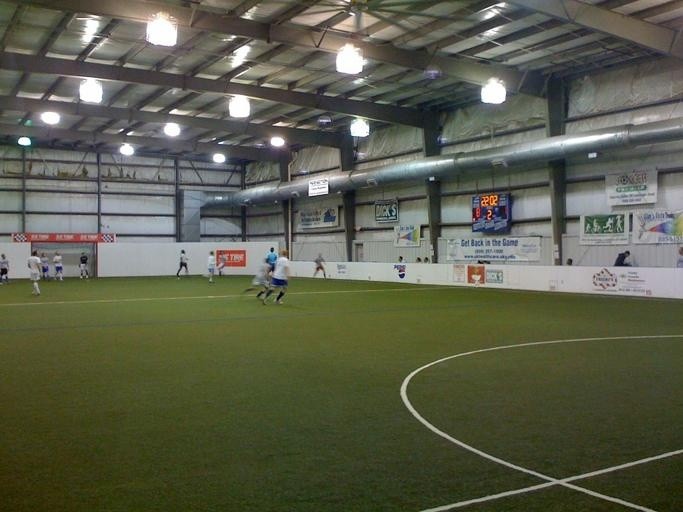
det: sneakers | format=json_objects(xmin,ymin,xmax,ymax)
[
  {"xmin": 0, "ymin": 282, "xmax": 3, "ymax": 285},
  {"xmin": 31, "ymin": 291, "xmax": 40, "ymax": 296},
  {"xmin": 261, "ymin": 297, "xmax": 267, "ymax": 306},
  {"xmin": 274, "ymin": 297, "xmax": 284, "ymax": 305}
]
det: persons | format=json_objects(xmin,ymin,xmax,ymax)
[
  {"xmin": 176, "ymin": 250, "xmax": 189, "ymax": 279},
  {"xmin": 613, "ymin": 251, "xmax": 630, "ymax": 266},
  {"xmin": 39, "ymin": 252, "xmax": 49, "ymax": 280},
  {"xmin": 78, "ymin": 253, "xmax": 88, "ymax": 279},
  {"xmin": 423, "ymin": 256, "xmax": 428, "ymax": 263},
  {"xmin": 264, "ymin": 246, "xmax": 277, "ymax": 274},
  {"xmin": 217, "ymin": 251, "xmax": 226, "ymax": 275},
  {"xmin": 312, "ymin": 252, "xmax": 326, "ymax": 280},
  {"xmin": 398, "ymin": 256, "xmax": 403, "ymax": 262},
  {"xmin": 262, "ymin": 250, "xmax": 290, "ymax": 305},
  {"xmin": 565, "ymin": 259, "xmax": 572, "ymax": 266},
  {"xmin": 676, "ymin": 247, "xmax": 683, "ymax": 268},
  {"xmin": 52, "ymin": 251, "xmax": 63, "ymax": 281},
  {"xmin": 0, "ymin": 254, "xmax": 8, "ymax": 283},
  {"xmin": 241, "ymin": 258, "xmax": 269, "ymax": 300},
  {"xmin": 26, "ymin": 250, "xmax": 41, "ymax": 295},
  {"xmin": 206, "ymin": 251, "xmax": 214, "ymax": 284},
  {"xmin": 415, "ymin": 257, "xmax": 421, "ymax": 263}
]
